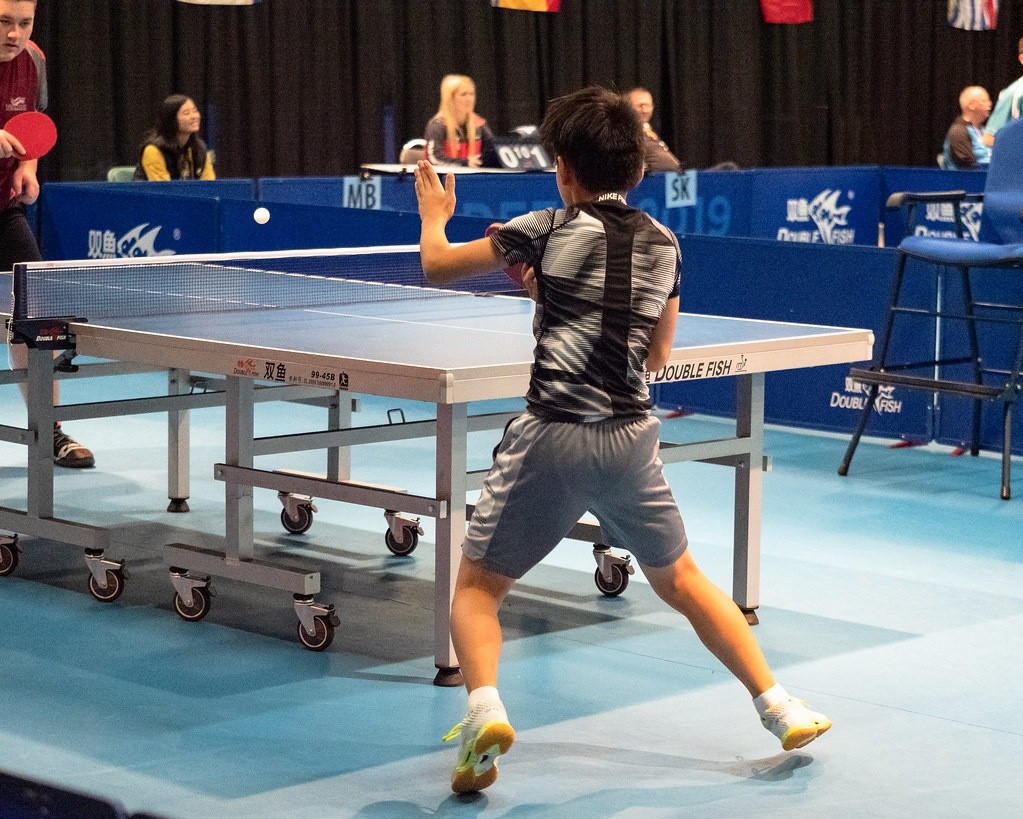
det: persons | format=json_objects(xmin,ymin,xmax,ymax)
[
  {"xmin": 425, "ymin": 74, "xmax": 497, "ymax": 169},
  {"xmin": 412, "ymin": 86, "xmax": 835, "ymax": 793},
  {"xmin": 625, "ymin": 89, "xmax": 742, "ymax": 171},
  {"xmin": 132, "ymin": 94, "xmax": 219, "ymax": 181},
  {"xmin": 942, "ymin": 84, "xmax": 994, "ymax": 170},
  {"xmin": 983, "ymin": 38, "xmax": 1022, "ymax": 147},
  {"xmin": 0, "ymin": 0, "xmax": 95, "ymax": 468}
]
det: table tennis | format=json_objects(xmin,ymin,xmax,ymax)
[{"xmin": 254, "ymin": 208, "xmax": 270, "ymax": 225}]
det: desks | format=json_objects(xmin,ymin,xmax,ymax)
[
  {"xmin": 361, "ymin": 161, "xmax": 525, "ymax": 174},
  {"xmin": 0, "ymin": 260, "xmax": 874, "ymax": 686}
]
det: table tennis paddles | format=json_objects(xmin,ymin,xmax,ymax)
[
  {"xmin": 3, "ymin": 111, "xmax": 58, "ymax": 162},
  {"xmin": 484, "ymin": 221, "xmax": 531, "ymax": 287}
]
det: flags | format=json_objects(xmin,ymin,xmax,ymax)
[
  {"xmin": 949, "ymin": 0, "xmax": 997, "ymax": 32},
  {"xmin": 764, "ymin": 0, "xmax": 816, "ymax": 24}
]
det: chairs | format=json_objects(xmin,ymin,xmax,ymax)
[{"xmin": 839, "ymin": 120, "xmax": 1023, "ymax": 500}]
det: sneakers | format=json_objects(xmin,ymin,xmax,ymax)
[
  {"xmin": 760, "ymin": 696, "xmax": 831, "ymax": 752},
  {"xmin": 54, "ymin": 421, "xmax": 96, "ymax": 468},
  {"xmin": 440, "ymin": 698, "xmax": 515, "ymax": 793}
]
